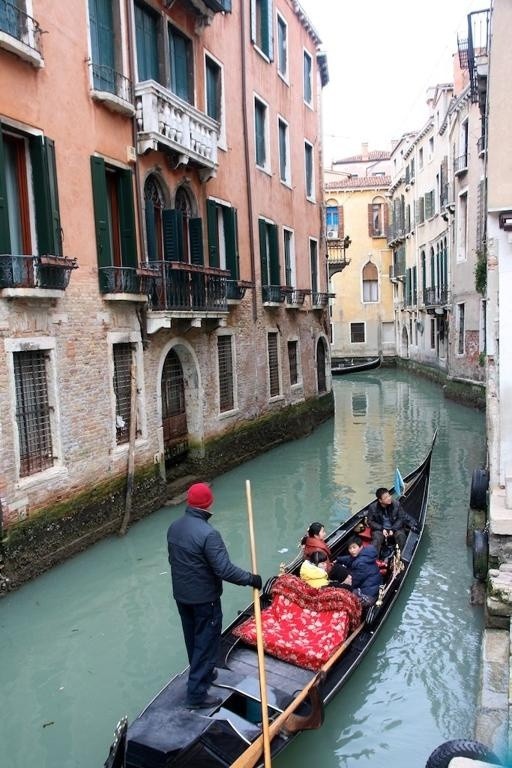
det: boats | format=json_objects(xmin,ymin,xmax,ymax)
[
  {"xmin": 332, "ymin": 358, "xmax": 380, "ymax": 376},
  {"xmin": 104, "ymin": 429, "xmax": 442, "ymax": 768}
]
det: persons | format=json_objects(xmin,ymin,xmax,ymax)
[
  {"xmin": 300, "ymin": 487, "xmax": 420, "ymax": 626},
  {"xmin": 165, "ymin": 482, "xmax": 263, "ymax": 710}
]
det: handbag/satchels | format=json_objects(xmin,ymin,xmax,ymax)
[{"xmin": 381, "ymin": 544, "xmax": 396, "ymax": 560}]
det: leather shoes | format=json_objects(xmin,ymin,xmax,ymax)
[
  {"xmin": 211, "ymin": 670, "xmax": 218, "ymax": 681},
  {"xmin": 186, "ymin": 694, "xmax": 223, "ymax": 709}
]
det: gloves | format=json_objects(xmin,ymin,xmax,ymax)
[{"xmin": 249, "ymin": 574, "xmax": 262, "ymax": 590}]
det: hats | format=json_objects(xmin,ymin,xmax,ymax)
[
  {"xmin": 328, "ymin": 563, "xmax": 349, "ymax": 583},
  {"xmin": 187, "ymin": 483, "xmax": 212, "ymax": 509}
]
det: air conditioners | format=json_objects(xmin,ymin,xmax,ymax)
[{"xmin": 327, "ymin": 231, "xmax": 339, "ymax": 238}]
